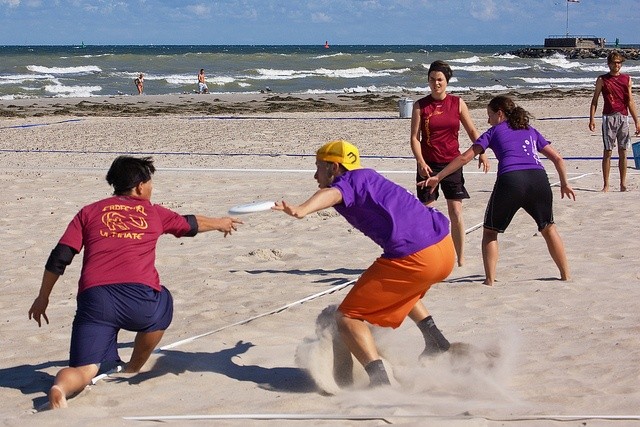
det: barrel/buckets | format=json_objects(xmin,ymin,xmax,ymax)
[
  {"xmin": 631, "ymin": 133, "xmax": 640, "ymax": 169},
  {"xmin": 399, "ymin": 100, "xmax": 414, "ymax": 118}
]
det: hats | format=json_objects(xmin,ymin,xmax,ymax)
[{"xmin": 316, "ymin": 140, "xmax": 364, "ymax": 170}]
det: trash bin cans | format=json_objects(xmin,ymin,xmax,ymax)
[{"xmin": 399, "ymin": 97, "xmax": 415, "ymax": 119}]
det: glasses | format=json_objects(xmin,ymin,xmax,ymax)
[{"xmin": 609, "ymin": 61, "xmax": 621, "ymax": 64}]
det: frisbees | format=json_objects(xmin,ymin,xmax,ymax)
[{"xmin": 228, "ymin": 201, "xmax": 276, "ymax": 215}]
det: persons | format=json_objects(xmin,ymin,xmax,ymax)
[
  {"xmin": 418, "ymin": 95, "xmax": 576, "ymax": 287},
  {"xmin": 588, "ymin": 50, "xmax": 640, "ymax": 192},
  {"xmin": 197, "ymin": 68, "xmax": 208, "ymax": 93},
  {"xmin": 28, "ymin": 154, "xmax": 244, "ymax": 412},
  {"xmin": 134, "ymin": 74, "xmax": 144, "ymax": 94},
  {"xmin": 410, "ymin": 60, "xmax": 490, "ymax": 267},
  {"xmin": 271, "ymin": 139, "xmax": 456, "ymax": 390}
]
